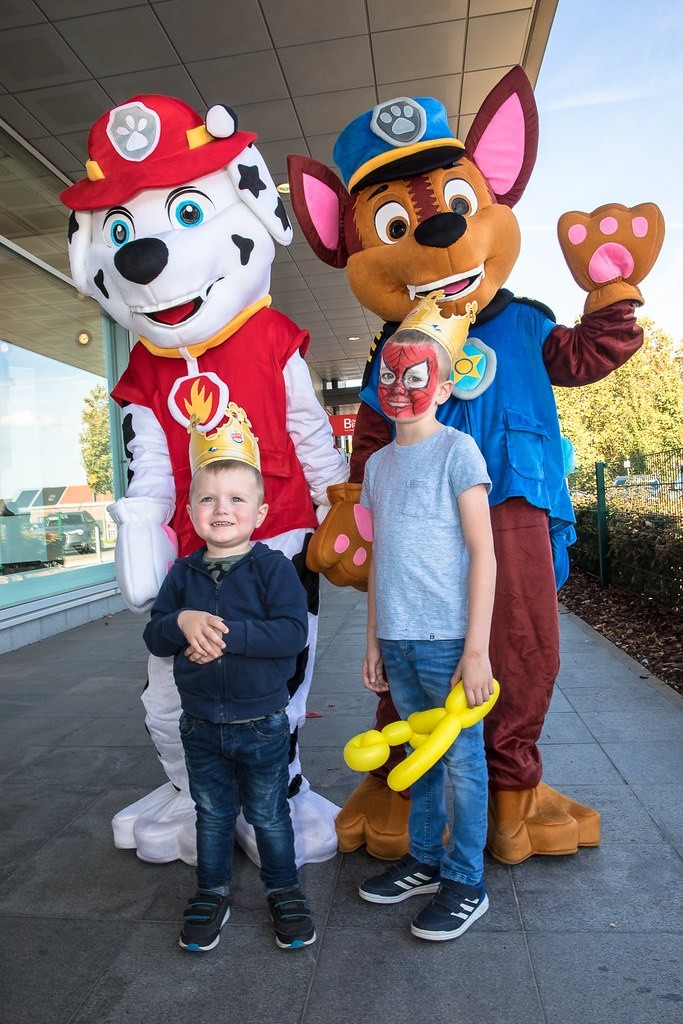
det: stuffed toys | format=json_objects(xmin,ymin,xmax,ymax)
[
  {"xmin": 284, "ymin": 66, "xmax": 665, "ymax": 872},
  {"xmin": 58, "ymin": 89, "xmax": 346, "ymax": 875}
]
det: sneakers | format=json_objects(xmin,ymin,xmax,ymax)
[
  {"xmin": 267, "ymin": 886, "xmax": 316, "ymax": 949},
  {"xmin": 359, "ymin": 852, "xmax": 443, "ymax": 904},
  {"xmin": 410, "ymin": 878, "xmax": 490, "ymax": 939},
  {"xmin": 179, "ymin": 890, "xmax": 230, "ymax": 952}
]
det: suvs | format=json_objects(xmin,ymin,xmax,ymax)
[{"xmin": 612, "ymin": 475, "xmax": 664, "ymax": 504}]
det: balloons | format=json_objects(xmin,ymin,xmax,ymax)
[{"xmin": 341, "ymin": 679, "xmax": 500, "ymax": 791}]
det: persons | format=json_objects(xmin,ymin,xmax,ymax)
[
  {"xmin": 143, "ymin": 462, "xmax": 315, "ymax": 952},
  {"xmin": 351, "ymin": 292, "xmax": 497, "ymax": 942}
]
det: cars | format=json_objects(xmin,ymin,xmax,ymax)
[{"xmin": 37, "ymin": 510, "xmax": 102, "ymax": 554}]
